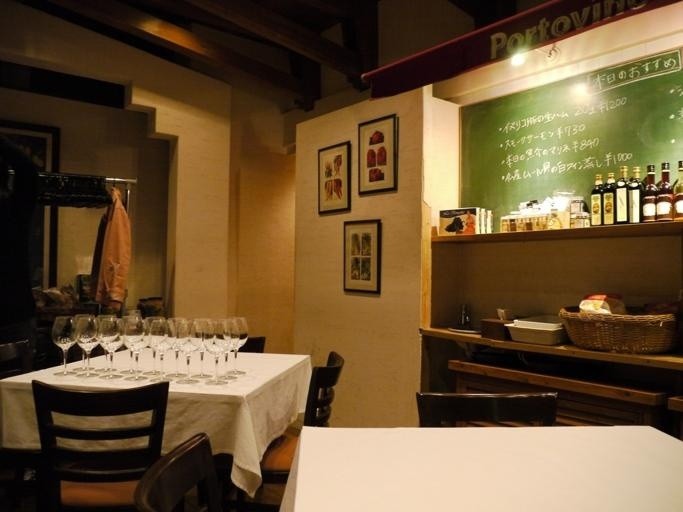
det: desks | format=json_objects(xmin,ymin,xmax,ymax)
[{"xmin": 275, "ymin": 423, "xmax": 682, "ymax": 512}]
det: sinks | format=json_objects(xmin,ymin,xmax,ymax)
[{"xmin": 448, "ymin": 324, "xmax": 484, "ymax": 335}]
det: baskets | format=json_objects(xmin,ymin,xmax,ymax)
[{"xmin": 558, "ymin": 305, "xmax": 682, "ymax": 352}]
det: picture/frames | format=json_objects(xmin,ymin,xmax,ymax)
[
  {"xmin": 315, "ymin": 141, "xmax": 353, "ymax": 215},
  {"xmin": 342, "ymin": 218, "xmax": 383, "ymax": 294},
  {"xmin": 0, "ymin": 117, "xmax": 62, "ymax": 293},
  {"xmin": 356, "ymin": 114, "xmax": 400, "ymax": 195}
]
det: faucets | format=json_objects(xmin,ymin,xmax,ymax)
[{"xmin": 457, "ymin": 303, "xmax": 472, "ymax": 326}]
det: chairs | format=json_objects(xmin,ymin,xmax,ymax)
[
  {"xmin": 130, "ymin": 432, "xmax": 276, "ymax": 511},
  {"xmin": 416, "ymin": 389, "xmax": 559, "ymax": 430},
  {"xmin": 0, "ymin": 336, "xmax": 34, "ymax": 381},
  {"xmin": 191, "ymin": 332, "xmax": 269, "ymax": 356},
  {"xmin": 31, "ymin": 376, "xmax": 170, "ymax": 512},
  {"xmin": 233, "ymin": 351, "xmax": 347, "ymax": 512}
]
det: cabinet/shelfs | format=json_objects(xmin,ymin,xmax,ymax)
[
  {"xmin": 419, "ymin": 221, "xmax": 682, "ymax": 429},
  {"xmin": 5, "ymin": 168, "xmax": 115, "ymax": 370}
]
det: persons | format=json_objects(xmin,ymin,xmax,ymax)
[{"xmin": 464, "ymin": 209, "xmax": 476, "ymax": 233}]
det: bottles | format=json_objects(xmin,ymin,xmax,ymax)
[
  {"xmin": 589, "ymin": 174, "xmax": 604, "ymax": 226},
  {"xmin": 630, "ymin": 165, "xmax": 645, "ymax": 223},
  {"xmin": 640, "ymin": 163, "xmax": 656, "ymax": 224},
  {"xmin": 614, "ymin": 165, "xmax": 629, "ymax": 223},
  {"xmin": 603, "ymin": 173, "xmax": 616, "ymax": 224},
  {"xmin": 656, "ymin": 162, "xmax": 675, "ymax": 223},
  {"xmin": 672, "ymin": 161, "xmax": 683, "ymax": 222}
]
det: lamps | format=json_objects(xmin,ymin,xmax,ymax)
[{"xmin": 507, "ymin": 43, "xmax": 564, "ymax": 68}]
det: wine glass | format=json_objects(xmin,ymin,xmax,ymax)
[
  {"xmin": 51, "ymin": 315, "xmax": 79, "ymax": 377},
  {"xmin": 72, "ymin": 313, "xmax": 250, "ymax": 387}
]
